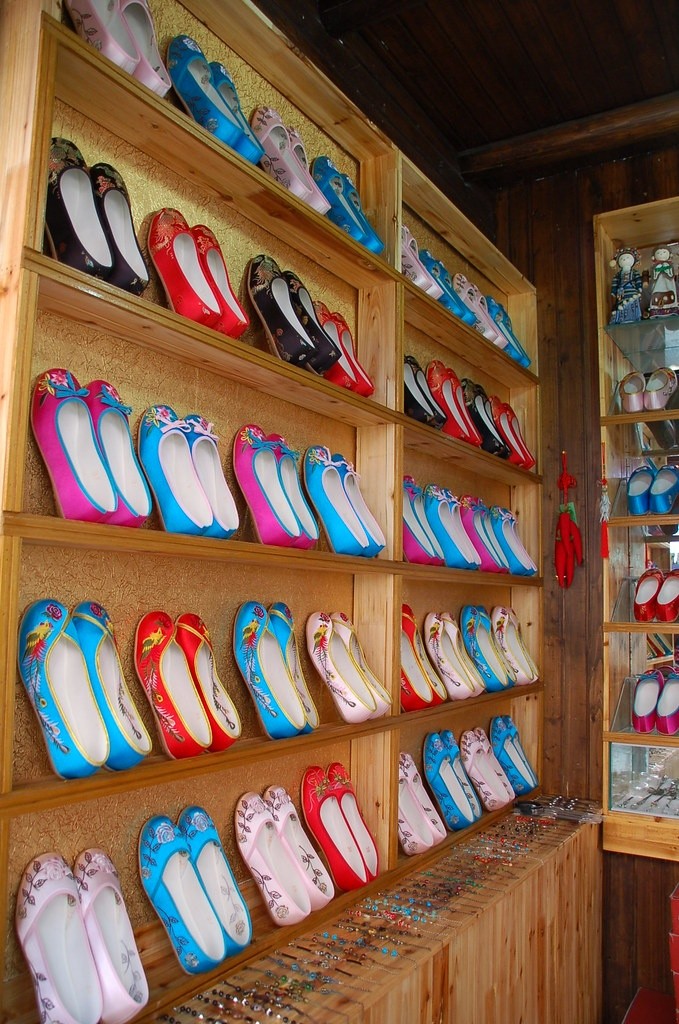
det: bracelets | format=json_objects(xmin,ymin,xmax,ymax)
[{"xmin": 154, "ymin": 795, "xmax": 609, "ymax": 1024}]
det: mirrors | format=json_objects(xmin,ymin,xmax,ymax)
[{"xmin": 620, "ymin": 242, "xmax": 679, "ymax": 783}]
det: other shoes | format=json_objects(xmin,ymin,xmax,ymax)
[
  {"xmin": 0, "ymin": 0, "xmax": 541, "ymax": 1024},
  {"xmin": 612, "ymin": 364, "xmax": 679, "ymax": 739}
]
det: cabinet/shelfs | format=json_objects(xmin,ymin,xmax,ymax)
[
  {"xmin": 0, "ymin": 0, "xmax": 547, "ymax": 1024},
  {"xmin": 592, "ymin": 194, "xmax": 679, "ymax": 865}
]
